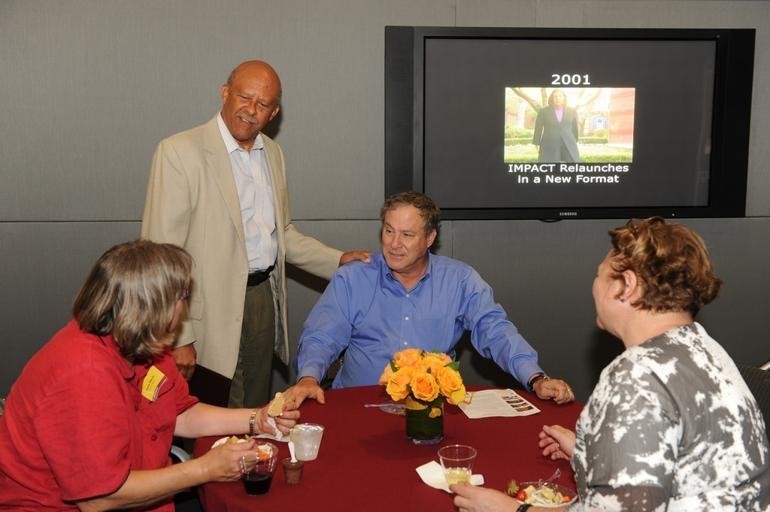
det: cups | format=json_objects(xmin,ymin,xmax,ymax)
[
  {"xmin": 282, "ymin": 457, "xmax": 305, "ymax": 485},
  {"xmin": 436, "ymin": 444, "xmax": 477, "ymax": 492},
  {"xmin": 290, "ymin": 422, "xmax": 327, "ymax": 462},
  {"xmin": 237, "ymin": 455, "xmax": 279, "ymax": 495}
]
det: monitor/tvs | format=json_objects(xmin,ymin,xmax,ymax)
[{"xmin": 381, "ymin": 24, "xmax": 757, "ymax": 222}]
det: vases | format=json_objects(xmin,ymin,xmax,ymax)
[{"xmin": 405, "ymin": 409, "xmax": 443, "ymax": 445}]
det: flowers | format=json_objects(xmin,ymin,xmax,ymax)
[{"xmin": 378, "ymin": 348, "xmax": 472, "ymax": 419}]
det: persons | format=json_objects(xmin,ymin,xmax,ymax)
[
  {"xmin": 449, "ymin": 215, "xmax": 770, "ymax": 512},
  {"xmin": 140, "ymin": 60, "xmax": 374, "ymax": 512},
  {"xmin": 0, "ymin": 240, "xmax": 301, "ymax": 512},
  {"xmin": 283, "ymin": 190, "xmax": 575, "ymax": 411},
  {"xmin": 532, "ymin": 90, "xmax": 580, "ymax": 162}
]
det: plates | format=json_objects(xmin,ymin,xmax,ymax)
[
  {"xmin": 210, "ymin": 440, "xmax": 279, "ymax": 462},
  {"xmin": 505, "ymin": 482, "xmax": 578, "ymax": 507},
  {"xmin": 381, "ymin": 398, "xmax": 407, "ymax": 417}
]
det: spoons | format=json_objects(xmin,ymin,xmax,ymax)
[{"xmin": 539, "ymin": 468, "xmax": 562, "ymax": 488}]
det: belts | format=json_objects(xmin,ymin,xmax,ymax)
[{"xmin": 246, "ymin": 265, "xmax": 274, "ymax": 287}]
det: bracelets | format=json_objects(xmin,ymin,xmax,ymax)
[
  {"xmin": 249, "ymin": 410, "xmax": 256, "ymax": 436},
  {"xmin": 528, "ymin": 374, "xmax": 548, "ymax": 392},
  {"xmin": 516, "ymin": 504, "xmax": 532, "ymax": 512}
]
